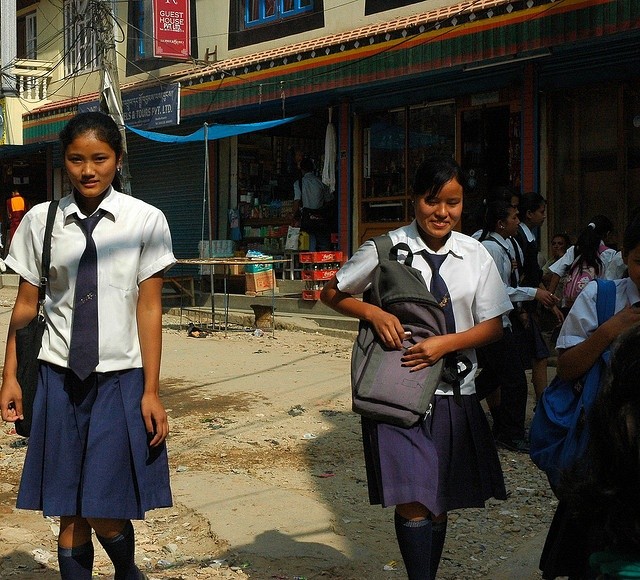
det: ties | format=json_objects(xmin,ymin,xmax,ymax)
[
  {"xmin": 70, "ymin": 214, "xmax": 105, "ymax": 380},
  {"xmin": 420, "ymin": 249, "xmax": 458, "ymax": 385}
]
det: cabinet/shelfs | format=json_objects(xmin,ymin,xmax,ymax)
[{"xmin": 239, "ymin": 216, "xmax": 299, "ymax": 273}]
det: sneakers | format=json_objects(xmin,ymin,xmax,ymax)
[{"xmin": 492, "ymin": 429, "xmax": 535, "ymax": 456}]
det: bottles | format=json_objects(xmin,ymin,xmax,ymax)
[
  {"xmin": 305, "ymin": 281, "xmax": 329, "ymax": 290},
  {"xmin": 303, "ymin": 263, "xmax": 339, "ymax": 270}
]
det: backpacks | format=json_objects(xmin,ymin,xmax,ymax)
[
  {"xmin": 350, "ymin": 232, "xmax": 446, "ymax": 429},
  {"xmin": 563, "ymin": 243, "xmax": 607, "ymax": 309},
  {"xmin": 528, "ymin": 279, "xmax": 618, "ymax": 484}
]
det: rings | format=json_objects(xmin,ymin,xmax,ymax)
[{"xmin": 550, "ymin": 294, "xmax": 553, "ymax": 297}]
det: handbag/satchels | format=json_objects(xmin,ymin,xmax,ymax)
[
  {"xmin": 300, "ymin": 208, "xmax": 325, "ymax": 232},
  {"xmin": 14, "ymin": 314, "xmax": 47, "ymax": 436}
]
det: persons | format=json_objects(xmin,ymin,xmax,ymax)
[
  {"xmin": 605, "ymin": 206, "xmax": 640, "ymax": 280},
  {"xmin": 541, "ymin": 234, "xmax": 572, "ymax": 331},
  {"xmin": 513, "ymin": 192, "xmax": 566, "ymax": 414},
  {"xmin": 0, "ymin": 111, "xmax": 172, "ymax": 580},
  {"xmin": 470, "ymin": 190, "xmax": 531, "ymax": 441},
  {"xmin": 6, "ymin": 188, "xmax": 32, "ymax": 241},
  {"xmin": 320, "ymin": 154, "xmax": 514, "ymax": 580},
  {"xmin": 477, "ymin": 201, "xmax": 561, "ymax": 454},
  {"xmin": 539, "ymin": 216, "xmax": 640, "ymax": 580},
  {"xmin": 284, "ymin": 158, "xmax": 332, "ymax": 251},
  {"xmin": 547, "ymin": 214, "xmax": 618, "ymax": 344}
]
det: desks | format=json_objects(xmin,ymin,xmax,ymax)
[
  {"xmin": 172, "ymin": 257, "xmax": 293, "ymax": 341},
  {"xmin": 162, "ymin": 275, "xmax": 195, "ymax": 308}
]
